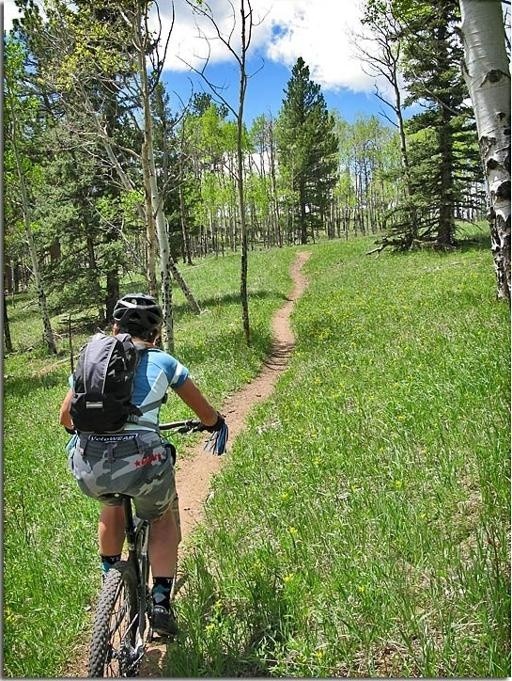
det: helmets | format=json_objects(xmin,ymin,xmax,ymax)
[{"xmin": 112, "ymin": 293, "xmax": 163, "ymax": 333}]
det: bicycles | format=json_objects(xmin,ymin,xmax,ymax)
[{"xmin": 84, "ymin": 418, "xmax": 201, "ymax": 678}]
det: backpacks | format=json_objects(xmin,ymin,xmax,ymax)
[{"xmin": 67, "ymin": 333, "xmax": 163, "ymax": 434}]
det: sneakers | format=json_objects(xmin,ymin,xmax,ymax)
[{"xmin": 151, "ymin": 604, "xmax": 178, "ymax": 639}]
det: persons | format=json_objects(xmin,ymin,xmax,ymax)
[{"xmin": 57, "ymin": 294, "xmax": 223, "ymax": 637}]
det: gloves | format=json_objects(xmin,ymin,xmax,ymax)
[{"xmin": 200, "ymin": 411, "xmax": 228, "ymax": 456}]
